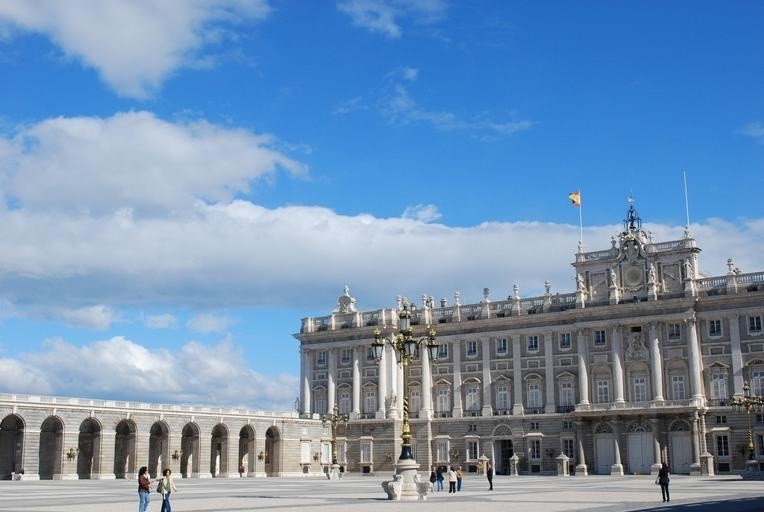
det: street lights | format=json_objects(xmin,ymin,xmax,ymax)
[
  {"xmin": 729, "ymin": 379, "xmax": 764, "ymax": 460},
  {"xmin": 322, "ymin": 403, "xmax": 348, "ymax": 464},
  {"xmin": 371, "ymin": 297, "xmax": 439, "ymax": 459}
]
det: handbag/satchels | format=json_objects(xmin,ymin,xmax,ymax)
[
  {"xmin": 157, "ymin": 479, "xmax": 164, "ymax": 492},
  {"xmin": 655, "ymin": 477, "xmax": 660, "ymax": 485}
]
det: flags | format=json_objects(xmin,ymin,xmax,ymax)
[{"xmin": 568, "ymin": 192, "xmax": 582, "ymax": 206}]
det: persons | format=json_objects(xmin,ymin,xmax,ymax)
[
  {"xmin": 447, "ymin": 466, "xmax": 462, "ymax": 494},
  {"xmin": 658, "ymin": 462, "xmax": 672, "ymax": 503},
  {"xmin": 430, "ymin": 467, "xmax": 444, "ymax": 493},
  {"xmin": 138, "ymin": 466, "xmax": 154, "ymax": 512},
  {"xmin": 487, "ymin": 464, "xmax": 493, "ymax": 491},
  {"xmin": 156, "ymin": 468, "xmax": 177, "ymax": 512}
]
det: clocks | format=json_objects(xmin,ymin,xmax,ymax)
[{"xmin": 623, "ymin": 262, "xmax": 644, "ymax": 288}]
